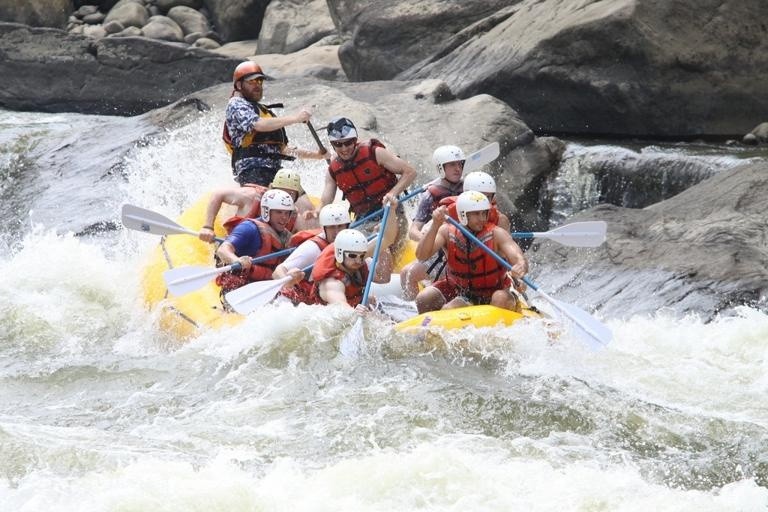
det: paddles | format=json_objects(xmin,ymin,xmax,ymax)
[
  {"xmin": 163, "ymin": 246, "xmax": 300, "ymax": 297},
  {"xmin": 443, "ymin": 213, "xmax": 613, "ymax": 347},
  {"xmin": 224, "ymin": 231, "xmax": 380, "ymax": 317},
  {"xmin": 347, "ymin": 140, "xmax": 501, "ymax": 229},
  {"xmin": 121, "ymin": 202, "xmax": 225, "ymax": 244},
  {"xmin": 339, "ymin": 199, "xmax": 391, "ymax": 357},
  {"xmin": 510, "ymin": 220, "xmax": 608, "ymax": 249}
]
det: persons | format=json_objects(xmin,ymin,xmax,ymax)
[
  {"xmin": 400, "ymin": 145, "xmax": 501, "ymax": 297},
  {"xmin": 416, "ymin": 190, "xmax": 529, "ymax": 316},
  {"xmin": 272, "ymin": 203, "xmax": 393, "ymax": 311},
  {"xmin": 308, "ymin": 193, "xmax": 398, "ymax": 320},
  {"xmin": 216, "ymin": 188, "xmax": 295, "ymax": 314},
  {"xmin": 198, "ymin": 168, "xmax": 304, "ymax": 288},
  {"xmin": 406, "ymin": 170, "xmax": 512, "ymax": 302},
  {"xmin": 302, "ymin": 116, "xmax": 417, "ymax": 253},
  {"xmin": 221, "ymin": 60, "xmax": 331, "ymax": 187}
]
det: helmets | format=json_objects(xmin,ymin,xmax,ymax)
[
  {"xmin": 433, "ymin": 145, "xmax": 467, "ymax": 181},
  {"xmin": 316, "ymin": 203, "xmax": 353, "ymax": 228},
  {"xmin": 456, "ymin": 191, "xmax": 494, "ymax": 227},
  {"xmin": 325, "ymin": 119, "xmax": 359, "ymax": 152},
  {"xmin": 233, "ymin": 60, "xmax": 277, "ymax": 93},
  {"xmin": 260, "ymin": 189, "xmax": 298, "ymax": 224},
  {"xmin": 272, "ymin": 167, "xmax": 303, "ymax": 202},
  {"xmin": 462, "ymin": 171, "xmax": 498, "ymax": 204},
  {"xmin": 332, "ymin": 230, "xmax": 371, "ymax": 265}
]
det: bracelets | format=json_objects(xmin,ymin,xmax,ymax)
[{"xmin": 203, "ymin": 225, "xmax": 215, "ymax": 232}]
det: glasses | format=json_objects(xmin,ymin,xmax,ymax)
[
  {"xmin": 343, "ymin": 251, "xmax": 368, "ymax": 263},
  {"xmin": 330, "ymin": 139, "xmax": 355, "ymax": 150},
  {"xmin": 244, "ymin": 78, "xmax": 267, "ymax": 88}
]
